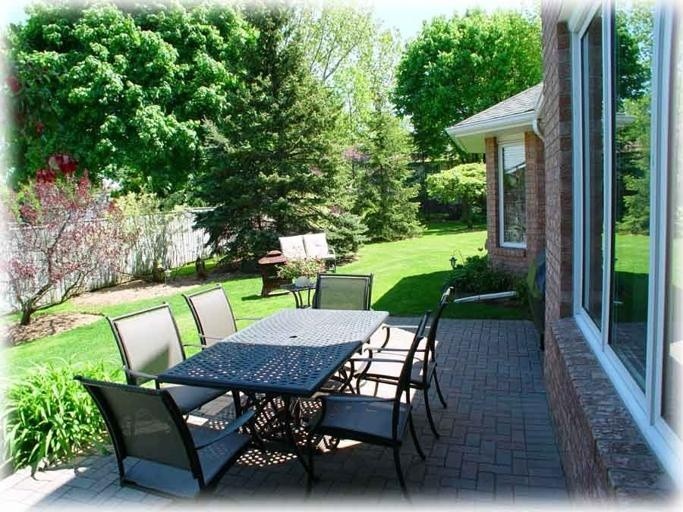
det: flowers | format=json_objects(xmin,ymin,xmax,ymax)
[{"xmin": 273, "ymin": 257, "xmax": 327, "ymax": 278}]
